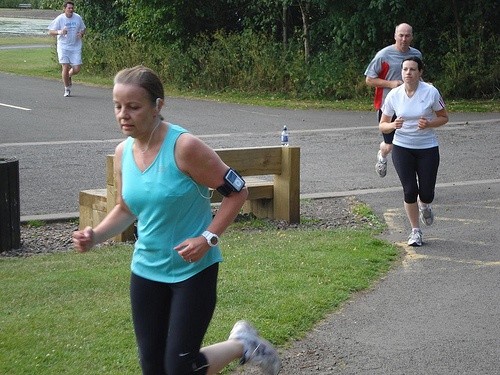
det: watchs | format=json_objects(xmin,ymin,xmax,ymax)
[{"xmin": 202, "ymin": 230, "xmax": 220, "ymax": 247}]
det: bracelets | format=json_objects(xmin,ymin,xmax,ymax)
[{"xmin": 57, "ymin": 30, "xmax": 62, "ymax": 35}]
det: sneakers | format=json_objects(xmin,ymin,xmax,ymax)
[
  {"xmin": 417, "ymin": 195, "xmax": 434, "ymax": 226},
  {"xmin": 228, "ymin": 319, "xmax": 281, "ymax": 375},
  {"xmin": 408, "ymin": 229, "xmax": 423, "ymax": 246},
  {"xmin": 375, "ymin": 150, "xmax": 387, "ymax": 178}
]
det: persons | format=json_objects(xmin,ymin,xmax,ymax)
[
  {"xmin": 52, "ymin": 0, "xmax": 86, "ymax": 97},
  {"xmin": 379, "ymin": 57, "xmax": 449, "ymax": 247},
  {"xmin": 72, "ymin": 63, "xmax": 283, "ymax": 375},
  {"xmin": 363, "ymin": 23, "xmax": 423, "ymax": 178}
]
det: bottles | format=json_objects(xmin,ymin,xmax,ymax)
[
  {"xmin": 281, "ymin": 126, "xmax": 289, "ymax": 146},
  {"xmin": 63, "ymin": 25, "xmax": 68, "ymax": 37}
]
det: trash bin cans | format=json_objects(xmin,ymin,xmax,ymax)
[{"xmin": 0, "ymin": 155, "xmax": 21, "ymax": 252}]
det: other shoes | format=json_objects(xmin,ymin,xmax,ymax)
[
  {"xmin": 69, "ymin": 77, "xmax": 71, "ymax": 86},
  {"xmin": 64, "ymin": 89, "xmax": 70, "ymax": 97}
]
